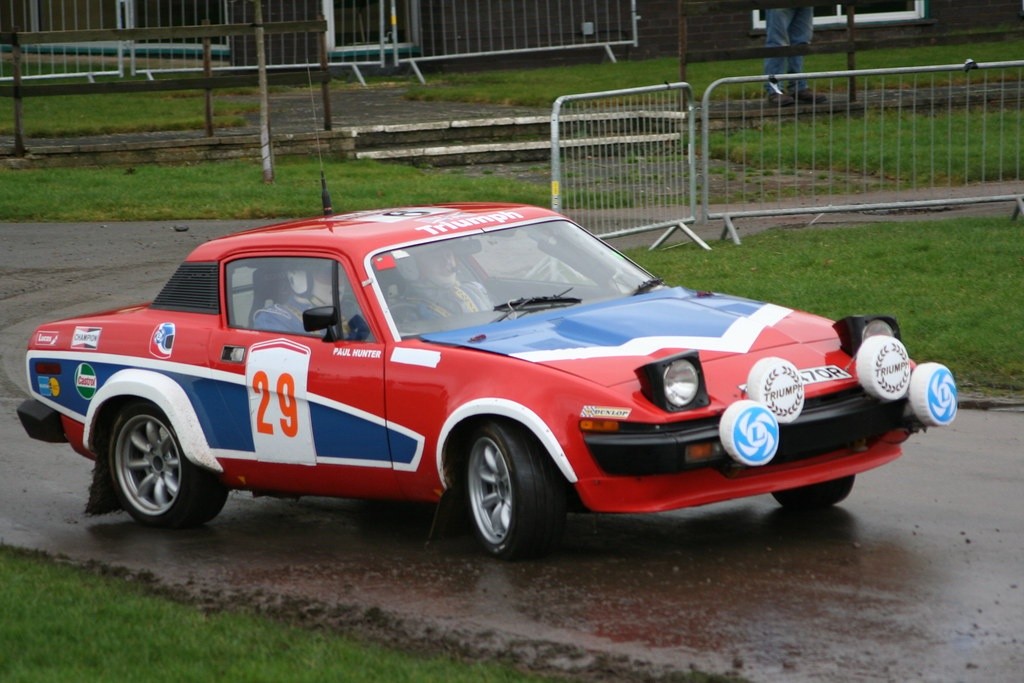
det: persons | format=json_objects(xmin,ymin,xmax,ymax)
[
  {"xmin": 401, "ymin": 242, "xmax": 493, "ymax": 322},
  {"xmin": 763, "ymin": 0, "xmax": 828, "ymax": 107},
  {"xmin": 253, "ymin": 257, "xmax": 375, "ymax": 341}
]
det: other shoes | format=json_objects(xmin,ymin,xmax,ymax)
[
  {"xmin": 792, "ymin": 88, "xmax": 826, "ymax": 103},
  {"xmin": 766, "ymin": 94, "xmax": 793, "ymax": 106}
]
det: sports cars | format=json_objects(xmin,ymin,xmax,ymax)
[{"xmin": 16, "ymin": 200, "xmax": 961, "ymax": 563}]
